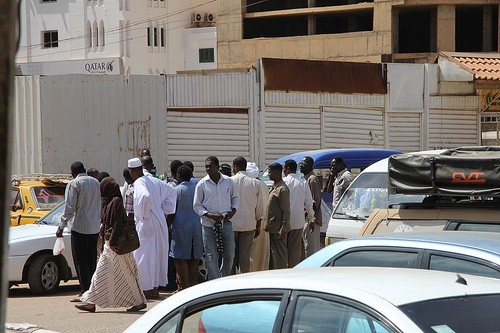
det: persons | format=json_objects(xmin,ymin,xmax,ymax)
[
  {"xmin": 75, "ymin": 177, "xmax": 148, "ymax": 313},
  {"xmin": 48, "ymin": 151, "xmax": 353, "ymax": 313},
  {"xmin": 192, "ymin": 155, "xmax": 240, "ymax": 282}
]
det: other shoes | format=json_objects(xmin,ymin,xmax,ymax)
[
  {"xmin": 144, "ymin": 290, "xmax": 159, "ymax": 299},
  {"xmin": 74, "ymin": 305, "xmax": 95, "ymax": 312},
  {"xmin": 70, "ymin": 296, "xmax": 82, "ymax": 302},
  {"xmin": 127, "ymin": 303, "xmax": 147, "ymax": 312}
]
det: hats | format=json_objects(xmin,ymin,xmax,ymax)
[{"xmin": 127, "ymin": 158, "xmax": 143, "ymax": 168}]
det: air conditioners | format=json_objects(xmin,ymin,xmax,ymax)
[
  {"xmin": 204, "ymin": 13, "xmax": 216, "ymax": 23},
  {"xmin": 191, "ymin": 13, "xmax": 204, "ymax": 23}
]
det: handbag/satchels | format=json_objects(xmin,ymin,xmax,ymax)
[
  {"xmin": 53, "ymin": 237, "xmax": 65, "ymax": 255},
  {"xmin": 114, "ymin": 216, "xmax": 140, "ymax": 255}
]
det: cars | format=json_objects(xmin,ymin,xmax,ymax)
[
  {"xmin": 120, "ymin": 266, "xmax": 500, "ymax": 333},
  {"xmin": 9, "ymin": 172, "xmax": 74, "ymax": 227},
  {"xmin": 8, "ymin": 201, "xmax": 78, "ymax": 296},
  {"xmin": 358, "ymin": 201, "xmax": 500, "ymax": 237},
  {"xmin": 196, "ymin": 231, "xmax": 500, "ymax": 333}
]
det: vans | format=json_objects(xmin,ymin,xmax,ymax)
[
  {"xmin": 257, "ymin": 148, "xmax": 406, "ymax": 213},
  {"xmin": 324, "ymin": 150, "xmax": 448, "ymax": 248}
]
